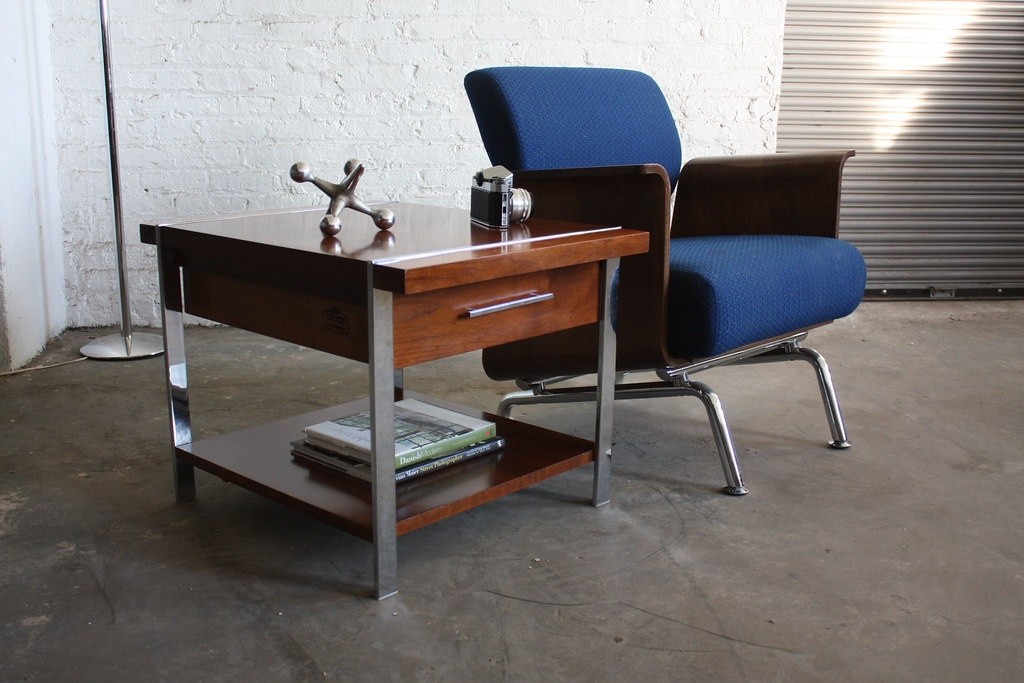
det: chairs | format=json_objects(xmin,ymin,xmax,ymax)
[{"xmin": 464, "ymin": 66, "xmax": 868, "ymax": 495}]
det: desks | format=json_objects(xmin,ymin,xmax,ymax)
[{"xmin": 138, "ymin": 202, "xmax": 651, "ymax": 601}]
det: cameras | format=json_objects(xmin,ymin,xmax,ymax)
[{"xmin": 470, "ymin": 165, "xmax": 531, "ymax": 229}]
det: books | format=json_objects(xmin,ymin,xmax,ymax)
[{"xmin": 287, "ymin": 395, "xmax": 505, "ymax": 488}]
